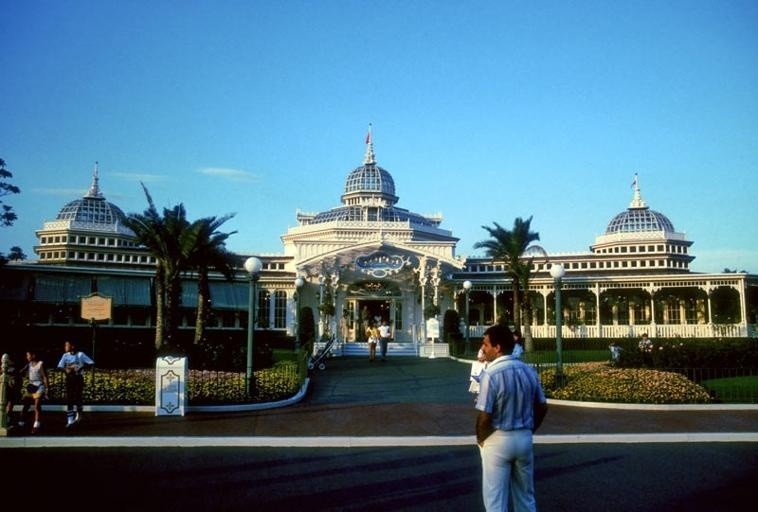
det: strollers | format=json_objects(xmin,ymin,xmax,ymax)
[{"xmin": 307, "ymin": 333, "xmax": 335, "ymax": 371}]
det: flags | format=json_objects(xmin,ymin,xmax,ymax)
[{"xmin": 366, "ymin": 131, "xmax": 371, "ymax": 144}]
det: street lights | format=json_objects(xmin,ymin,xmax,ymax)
[
  {"xmin": 295, "ymin": 279, "xmax": 303, "ymax": 353},
  {"xmin": 244, "ymin": 257, "xmax": 262, "ymax": 397},
  {"xmin": 550, "ymin": 264, "xmax": 566, "ymax": 385},
  {"xmin": 463, "ymin": 280, "xmax": 472, "ymax": 355}
]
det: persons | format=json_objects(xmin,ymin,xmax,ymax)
[
  {"xmin": 473, "ymin": 341, "xmax": 488, "ymax": 407},
  {"xmin": 379, "ymin": 320, "xmax": 391, "ymax": 360},
  {"xmin": 607, "ymin": 342, "xmax": 626, "ymax": 363},
  {"xmin": 359, "ymin": 305, "xmax": 370, "ymax": 342},
  {"xmin": 637, "ymin": 333, "xmax": 654, "ymax": 355},
  {"xmin": 17, "ymin": 350, "xmax": 50, "ymax": 430},
  {"xmin": 56, "ymin": 339, "xmax": 94, "ymax": 428},
  {"xmin": 373, "ymin": 311, "xmax": 384, "ymax": 326},
  {"xmin": 476, "ymin": 324, "xmax": 549, "ymax": 512},
  {"xmin": 511, "ymin": 330, "xmax": 524, "ymax": 360},
  {"xmin": 0, "ymin": 357, "xmax": 16, "ymax": 430},
  {"xmin": 365, "ymin": 319, "xmax": 379, "ymax": 362}
]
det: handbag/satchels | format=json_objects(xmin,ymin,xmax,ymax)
[{"xmin": 27, "ymin": 384, "xmax": 38, "ymax": 394}]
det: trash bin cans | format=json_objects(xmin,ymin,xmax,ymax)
[{"xmin": 155, "ymin": 355, "xmax": 189, "ymax": 417}]
